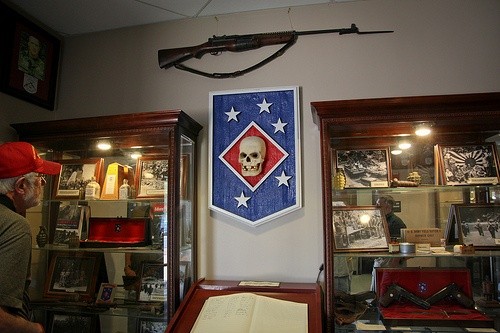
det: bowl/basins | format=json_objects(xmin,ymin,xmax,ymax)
[
  {"xmin": 121, "ymin": 275, "xmax": 138, "ymax": 290},
  {"xmin": 399, "ymin": 244, "xmax": 416, "ymax": 254}
]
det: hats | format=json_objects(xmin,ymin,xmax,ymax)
[
  {"xmin": 477, "ymin": 219, "xmax": 480, "ymax": 222},
  {"xmin": 462, "ymin": 221, "xmax": 465, "ymax": 223},
  {"xmin": 0, "ymin": 142, "xmax": 61, "ymax": 179},
  {"xmin": 489, "ymin": 219, "xmax": 494, "ymax": 221}
]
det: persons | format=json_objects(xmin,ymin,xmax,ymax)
[
  {"xmin": 462, "ymin": 219, "xmax": 498, "ymax": 238},
  {"xmin": 0, "ymin": 142, "xmax": 62, "ymax": 333},
  {"xmin": 352, "ymin": 160, "xmax": 363, "ymax": 170},
  {"xmin": 371, "ymin": 196, "xmax": 407, "ymax": 291},
  {"xmin": 144, "ymin": 280, "xmax": 163, "ymax": 295},
  {"xmin": 19, "ymin": 34, "xmax": 44, "ymax": 80},
  {"xmin": 334, "ymin": 212, "xmax": 383, "ymax": 247},
  {"xmin": 443, "ymin": 147, "xmax": 493, "ymax": 183},
  {"xmin": 334, "ymin": 256, "xmax": 353, "ymax": 294}
]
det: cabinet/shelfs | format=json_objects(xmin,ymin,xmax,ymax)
[
  {"xmin": 311, "ymin": 90, "xmax": 500, "ymax": 333},
  {"xmin": 8, "ymin": 110, "xmax": 203, "ymax": 333}
]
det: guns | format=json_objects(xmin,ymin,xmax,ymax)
[
  {"xmin": 157, "ymin": 23, "xmax": 395, "ymax": 71},
  {"xmin": 380, "ymin": 283, "xmax": 431, "ymax": 310},
  {"xmin": 425, "ymin": 283, "xmax": 475, "ymax": 307}
]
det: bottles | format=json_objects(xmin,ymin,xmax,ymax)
[
  {"xmin": 118, "ymin": 179, "xmax": 131, "ymax": 200},
  {"xmin": 35, "ymin": 225, "xmax": 47, "ymax": 248},
  {"xmin": 407, "ymin": 172, "xmax": 422, "ymax": 186},
  {"xmin": 334, "ymin": 168, "xmax": 346, "ymax": 190},
  {"xmin": 85, "ymin": 176, "xmax": 100, "ymax": 201}
]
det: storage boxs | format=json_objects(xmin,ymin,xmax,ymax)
[
  {"xmin": 374, "ymin": 266, "xmax": 495, "ymax": 326},
  {"xmin": 400, "ymin": 228, "xmax": 442, "ymax": 247},
  {"xmin": 79, "ymin": 215, "xmax": 153, "ymax": 249}
]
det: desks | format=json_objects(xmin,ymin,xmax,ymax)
[{"xmin": 166, "ymin": 278, "xmax": 324, "ymax": 333}]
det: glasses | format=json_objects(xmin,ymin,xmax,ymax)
[{"xmin": 35, "ymin": 172, "xmax": 45, "ymax": 180}]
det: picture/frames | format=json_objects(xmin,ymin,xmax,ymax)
[
  {"xmin": 332, "ymin": 146, "xmax": 391, "ymax": 189},
  {"xmin": 134, "ymin": 153, "xmax": 190, "ymax": 197},
  {"xmin": 98, "ymin": 282, "xmax": 117, "ymax": 303},
  {"xmin": 44, "ymin": 251, "xmax": 102, "ymax": 299},
  {"xmin": 333, "ymin": 206, "xmax": 391, "ymax": 253},
  {"xmin": 53, "ymin": 157, "xmax": 105, "ymax": 200},
  {"xmin": 433, "ymin": 141, "xmax": 500, "ymax": 250},
  {"xmin": 127, "ymin": 307, "xmax": 167, "ymax": 333},
  {"xmin": 45, "ymin": 310, "xmax": 98, "ymax": 333},
  {"xmin": 0, "ymin": 0, "xmax": 65, "ymax": 112},
  {"xmin": 140, "ymin": 262, "xmax": 191, "ymax": 302}
]
